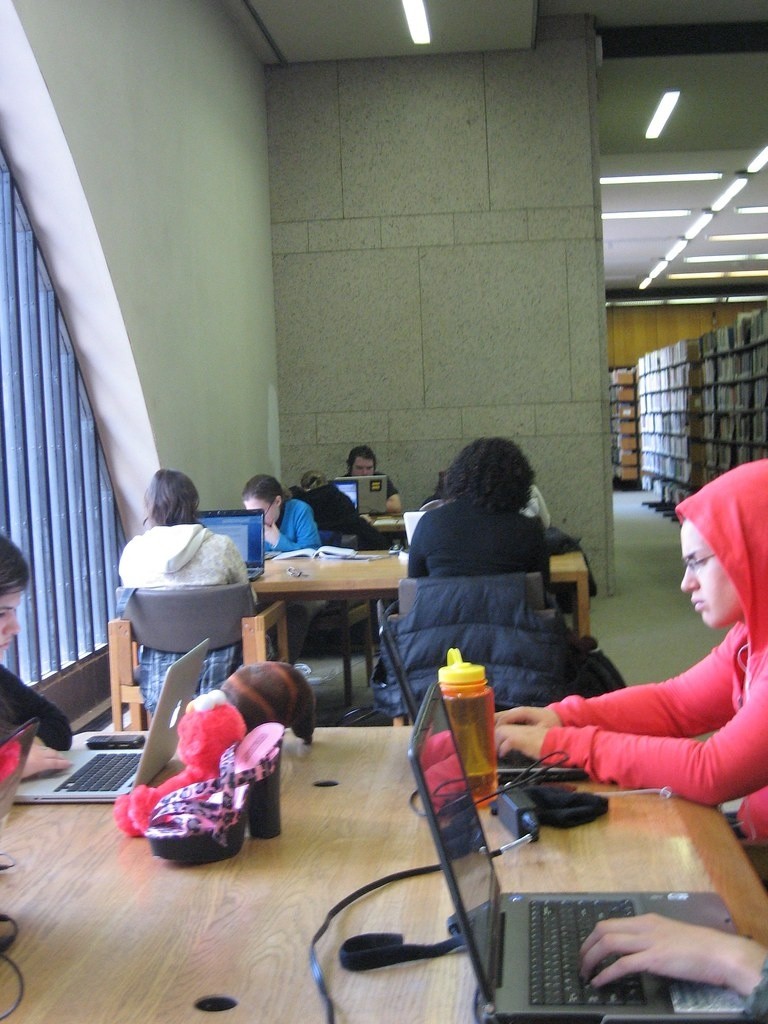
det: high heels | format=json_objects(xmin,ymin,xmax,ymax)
[{"xmin": 145, "ymin": 721, "xmax": 285, "ymax": 863}]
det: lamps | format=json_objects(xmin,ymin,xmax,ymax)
[
  {"xmin": 600, "ymin": 90, "xmax": 768, "ymax": 306},
  {"xmin": 401, "ymin": 0, "xmax": 433, "ymax": 45}
]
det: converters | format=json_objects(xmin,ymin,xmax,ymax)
[{"xmin": 497, "ymin": 784, "xmax": 539, "ymax": 844}]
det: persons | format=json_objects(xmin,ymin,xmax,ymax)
[
  {"xmin": 408, "ymin": 438, "xmax": 549, "ymax": 592},
  {"xmin": 418, "ymin": 729, "xmax": 468, "ymax": 815},
  {"xmin": 243, "ymin": 474, "xmax": 328, "ymax": 666},
  {"xmin": 341, "ymin": 446, "xmax": 401, "ymax": 516},
  {"xmin": 578, "ymin": 913, "xmax": 768, "ymax": 1024},
  {"xmin": 491, "ymin": 459, "xmax": 768, "ymax": 889},
  {"xmin": 0, "ymin": 535, "xmax": 73, "ymax": 780},
  {"xmin": 117, "ymin": 468, "xmax": 256, "ymax": 663},
  {"xmin": 290, "ymin": 471, "xmax": 381, "ymax": 657}
]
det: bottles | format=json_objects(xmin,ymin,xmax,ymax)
[{"xmin": 437, "ymin": 648, "xmax": 499, "ymax": 808}]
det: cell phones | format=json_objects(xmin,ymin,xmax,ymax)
[{"xmin": 85, "ymin": 735, "xmax": 145, "ymax": 750}]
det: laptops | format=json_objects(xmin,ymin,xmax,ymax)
[
  {"xmin": 193, "ymin": 509, "xmax": 264, "ymax": 581},
  {"xmin": 336, "ymin": 475, "xmax": 388, "ymax": 514},
  {"xmin": 407, "ymin": 682, "xmax": 747, "ymax": 1024},
  {"xmin": 328, "ymin": 479, "xmax": 358, "ymax": 512},
  {"xmin": 403, "ymin": 511, "xmax": 426, "ymax": 545},
  {"xmin": 14, "ymin": 638, "xmax": 210, "ymax": 802},
  {"xmin": 377, "ymin": 600, "xmax": 589, "ymax": 784}
]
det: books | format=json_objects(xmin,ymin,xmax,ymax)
[{"xmin": 608, "ymin": 308, "xmax": 768, "ymax": 506}]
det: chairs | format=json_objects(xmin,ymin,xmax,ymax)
[{"xmin": 108, "ymin": 473, "xmax": 627, "ymax": 732}]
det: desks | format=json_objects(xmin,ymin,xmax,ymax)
[
  {"xmin": 368, "ymin": 510, "xmax": 412, "ymax": 549},
  {"xmin": 1, "ymin": 736, "xmax": 768, "ymax": 1024},
  {"xmin": 250, "ymin": 548, "xmax": 590, "ymax": 706}
]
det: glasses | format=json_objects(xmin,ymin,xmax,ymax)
[{"xmin": 686, "ymin": 553, "xmax": 715, "ymax": 575}]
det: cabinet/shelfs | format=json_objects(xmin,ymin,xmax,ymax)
[
  {"xmin": 704, "ymin": 314, "xmax": 768, "ymax": 485},
  {"xmin": 638, "ymin": 340, "xmax": 704, "ymax": 522},
  {"xmin": 610, "ymin": 368, "xmax": 640, "ymax": 489}
]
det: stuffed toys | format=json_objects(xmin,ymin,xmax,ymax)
[{"xmin": 115, "ymin": 661, "xmax": 315, "ymax": 837}]
